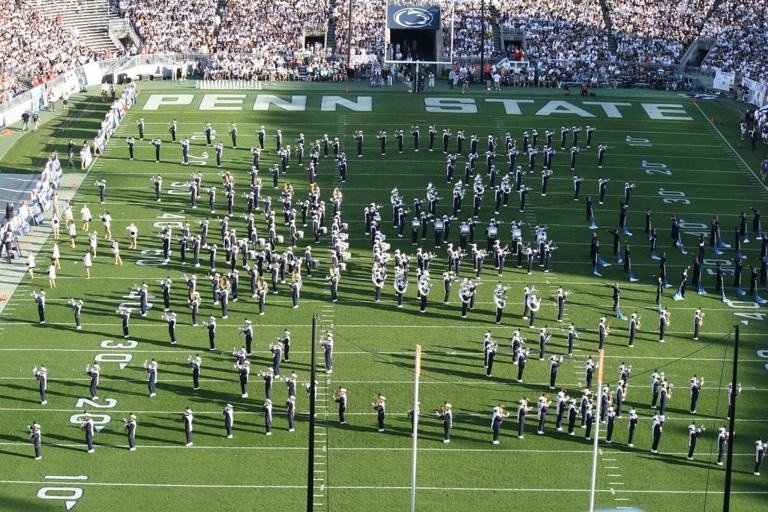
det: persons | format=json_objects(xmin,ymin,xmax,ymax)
[
  {"xmin": 1, "ymin": 0, "xmax": 767, "ymax": 152},
  {"xmin": 1, "ymin": 115, "xmax": 767, "ymax": 476}
]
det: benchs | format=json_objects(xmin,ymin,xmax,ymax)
[{"xmin": 23, "ymin": 0, "xmax": 128, "ymax": 57}]
